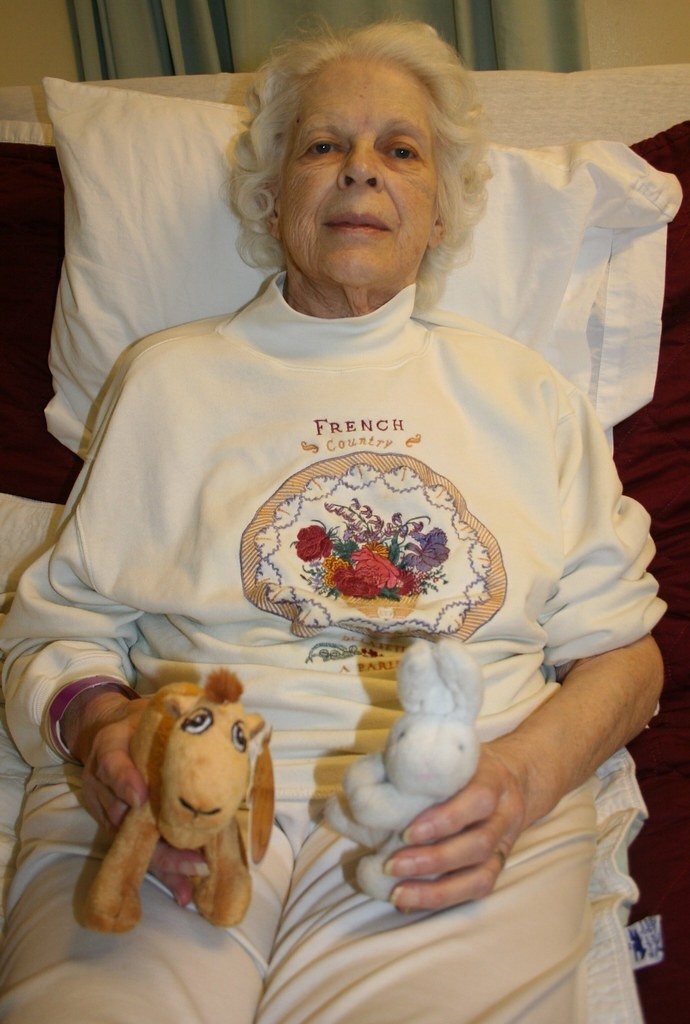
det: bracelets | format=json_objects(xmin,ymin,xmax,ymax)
[{"xmin": 50, "ymin": 675, "xmax": 141, "ymax": 768}]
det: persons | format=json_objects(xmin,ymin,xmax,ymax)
[{"xmin": 0, "ymin": 17, "xmax": 663, "ymax": 1024}]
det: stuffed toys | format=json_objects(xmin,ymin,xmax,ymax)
[
  {"xmin": 325, "ymin": 638, "xmax": 483, "ymax": 901},
  {"xmin": 85, "ymin": 671, "xmax": 275, "ymax": 934}
]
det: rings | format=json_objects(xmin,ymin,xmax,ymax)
[{"xmin": 492, "ymin": 848, "xmax": 506, "ymax": 869}]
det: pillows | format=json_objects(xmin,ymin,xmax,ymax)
[{"xmin": 41, "ymin": 70, "xmax": 682, "ymax": 460}]
